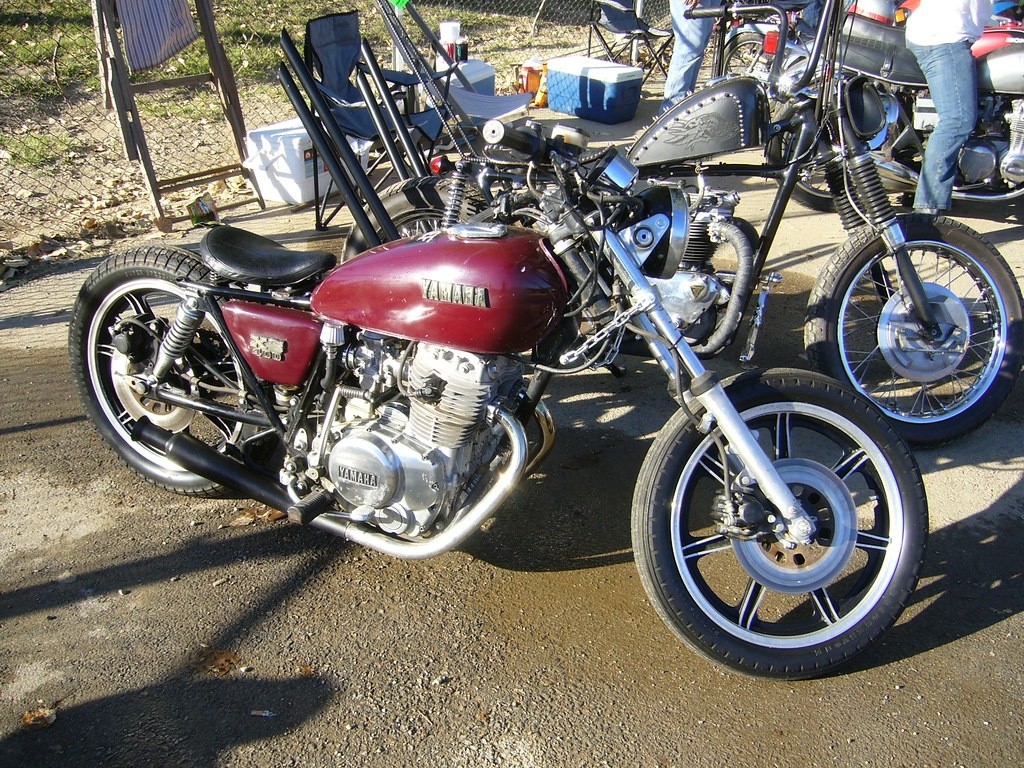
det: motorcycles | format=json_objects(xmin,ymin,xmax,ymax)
[
  {"xmin": 721, "ymin": 0, "xmax": 1024, "ymax": 214},
  {"xmin": 278, "ymin": 0, "xmax": 1024, "ymax": 453},
  {"xmin": 69, "ymin": 121, "xmax": 929, "ymax": 680}
]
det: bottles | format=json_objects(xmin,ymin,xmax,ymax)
[
  {"xmin": 536, "ymin": 82, "xmax": 547, "ymax": 107},
  {"xmin": 539, "ymin": 63, "xmax": 547, "ymax": 87},
  {"xmin": 517, "ymin": 74, "xmax": 525, "ymax": 94}
]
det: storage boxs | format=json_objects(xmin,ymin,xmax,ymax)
[
  {"xmin": 547, "ymin": 54, "xmax": 644, "ymax": 125},
  {"xmin": 242, "ymin": 117, "xmax": 358, "ymax": 205},
  {"xmin": 418, "ymin": 59, "xmax": 495, "ymax": 129}
]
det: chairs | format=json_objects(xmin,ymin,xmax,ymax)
[
  {"xmin": 303, "ymin": 9, "xmax": 459, "ymax": 232},
  {"xmin": 587, "ymin": 0, "xmax": 674, "ymax": 100}
]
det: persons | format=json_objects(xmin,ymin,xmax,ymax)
[
  {"xmin": 905, "ymin": 0, "xmax": 994, "ymax": 215},
  {"xmin": 658, "ymin": 0, "xmax": 722, "ymax": 118}
]
